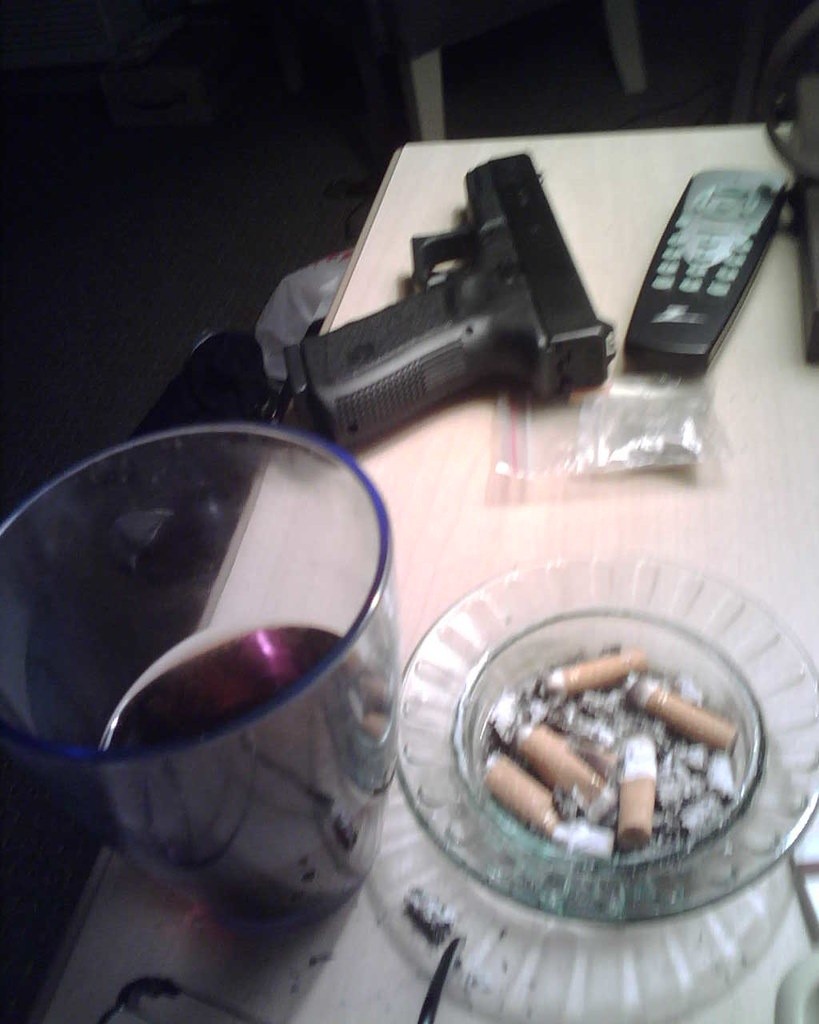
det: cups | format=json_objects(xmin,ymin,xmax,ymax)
[{"xmin": 1, "ymin": 421, "xmax": 403, "ymax": 938}]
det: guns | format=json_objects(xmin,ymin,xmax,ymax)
[{"xmin": 279, "ymin": 152, "xmax": 619, "ymax": 448}]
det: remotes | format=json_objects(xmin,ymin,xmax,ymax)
[{"xmin": 624, "ymin": 168, "xmax": 787, "ymax": 370}]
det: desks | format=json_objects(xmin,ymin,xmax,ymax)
[{"xmin": 34, "ymin": 124, "xmax": 819, "ymax": 1023}]
